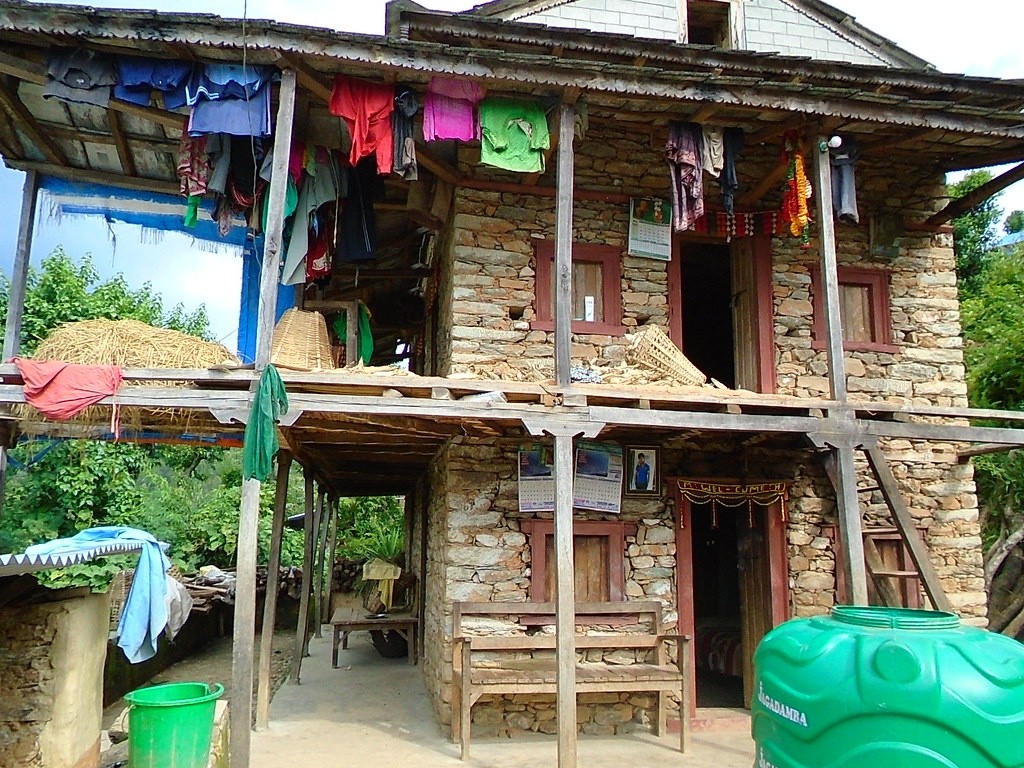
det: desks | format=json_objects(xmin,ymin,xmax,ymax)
[{"xmin": 330, "ymin": 608, "xmax": 419, "ymax": 669}]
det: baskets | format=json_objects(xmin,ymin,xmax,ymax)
[
  {"xmin": 624, "ymin": 324, "xmax": 708, "ymax": 387},
  {"xmin": 268, "ymin": 305, "xmax": 336, "ymax": 372}
]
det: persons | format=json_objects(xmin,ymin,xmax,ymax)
[{"xmin": 636, "ymin": 453, "xmax": 650, "ymax": 490}]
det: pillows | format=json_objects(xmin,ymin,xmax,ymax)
[{"xmin": 697, "ymin": 616, "xmax": 741, "ymax": 629}]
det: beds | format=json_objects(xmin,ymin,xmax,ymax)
[{"xmin": 696, "ymin": 616, "xmax": 742, "ymax": 686}]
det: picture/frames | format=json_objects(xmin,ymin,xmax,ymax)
[{"xmin": 622, "ymin": 443, "xmax": 663, "ymax": 499}]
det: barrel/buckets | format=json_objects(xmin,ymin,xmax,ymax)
[{"xmin": 124, "ymin": 682, "xmax": 224, "ymax": 768}]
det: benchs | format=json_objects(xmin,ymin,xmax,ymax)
[{"xmin": 450, "ymin": 601, "xmax": 692, "ymax": 761}]
